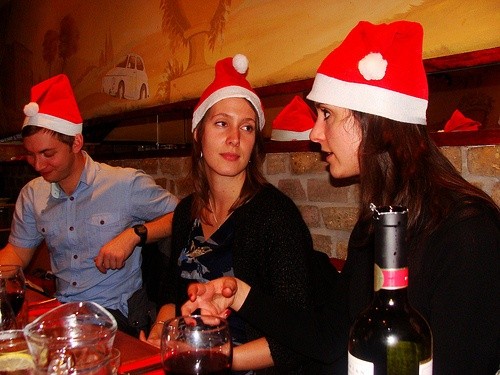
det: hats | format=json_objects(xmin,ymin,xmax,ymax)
[
  {"xmin": 307, "ymin": 21, "xmax": 428, "ymax": 125},
  {"xmin": 22, "ymin": 74, "xmax": 83, "ymax": 136},
  {"xmin": 437, "ymin": 109, "xmax": 478, "ymax": 132},
  {"xmin": 271, "ymin": 96, "xmax": 318, "ymax": 141},
  {"xmin": 193, "ymin": 54, "xmax": 266, "ymax": 131}
]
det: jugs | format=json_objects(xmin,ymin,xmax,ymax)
[{"xmin": 23, "ymin": 300, "xmax": 121, "ymax": 375}]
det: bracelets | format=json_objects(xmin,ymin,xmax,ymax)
[{"xmin": 150, "ymin": 321, "xmax": 164, "ymax": 329}]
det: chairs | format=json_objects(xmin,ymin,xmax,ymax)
[{"xmin": 139, "ymin": 234, "xmax": 170, "ymax": 330}]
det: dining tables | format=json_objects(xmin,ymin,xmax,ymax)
[{"xmin": 0, "ymin": 290, "xmax": 172, "ymax": 375}]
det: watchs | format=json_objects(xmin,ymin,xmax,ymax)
[{"xmin": 131, "ymin": 224, "xmax": 149, "ymax": 247}]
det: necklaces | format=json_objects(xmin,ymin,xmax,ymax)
[{"xmin": 208, "ymin": 199, "xmax": 219, "ymax": 226}]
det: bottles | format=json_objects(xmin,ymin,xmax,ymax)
[
  {"xmin": 346, "ymin": 205, "xmax": 434, "ymax": 375},
  {"xmin": 0, "ymin": 273, "xmax": 17, "ymax": 329}
]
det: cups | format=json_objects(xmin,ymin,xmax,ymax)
[
  {"xmin": 160, "ymin": 314, "xmax": 233, "ymax": 375},
  {"xmin": 0, "ymin": 329, "xmax": 31, "ymax": 375},
  {"xmin": 0, "ymin": 264, "xmax": 26, "ymax": 317}
]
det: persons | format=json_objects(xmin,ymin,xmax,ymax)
[
  {"xmin": 133, "ymin": 57, "xmax": 312, "ymax": 351},
  {"xmin": 0, "ymin": 74, "xmax": 180, "ymax": 335},
  {"xmin": 227, "ymin": 19, "xmax": 500, "ymax": 375}
]
